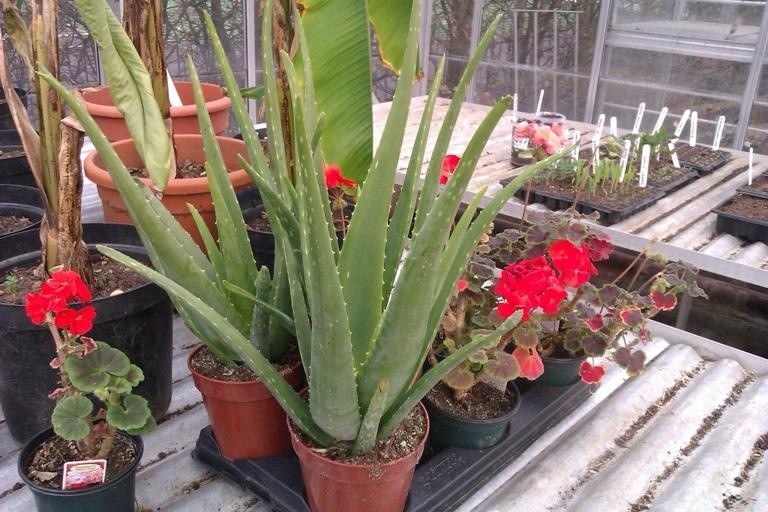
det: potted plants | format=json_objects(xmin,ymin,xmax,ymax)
[
  {"xmin": 498, "ymin": 132, "xmax": 731, "ymax": 220},
  {"xmin": 427, "ymin": 182, "xmax": 703, "ymax": 450},
  {"xmin": 0, "ymin": 5, "xmax": 564, "ymax": 509},
  {"xmin": 713, "ymin": 167, "xmax": 768, "ymax": 241}
]
